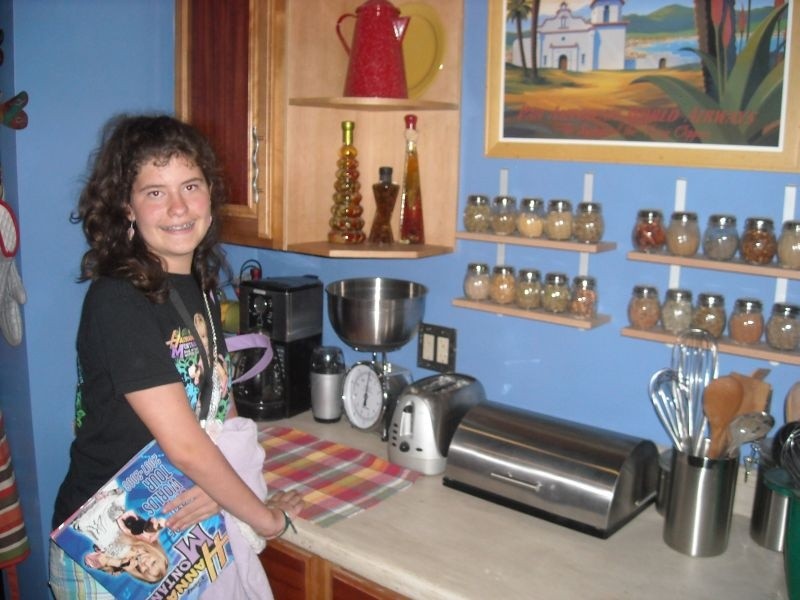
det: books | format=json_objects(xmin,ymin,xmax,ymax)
[{"xmin": 50, "ymin": 439, "xmax": 235, "ymax": 600}]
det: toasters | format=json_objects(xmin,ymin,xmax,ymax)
[{"xmin": 387, "ymin": 372, "xmax": 487, "ymax": 475}]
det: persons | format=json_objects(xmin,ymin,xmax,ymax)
[
  {"xmin": 72, "ymin": 478, "xmax": 168, "ymax": 583},
  {"xmin": 48, "ymin": 114, "xmax": 306, "ymax": 600}
]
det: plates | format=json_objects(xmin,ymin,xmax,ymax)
[{"xmin": 396, "ymin": 0, "xmax": 447, "ymax": 100}]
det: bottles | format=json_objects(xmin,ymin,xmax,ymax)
[
  {"xmin": 327, "ymin": 113, "xmax": 426, "ymax": 246},
  {"xmin": 462, "ymin": 263, "xmax": 597, "ymax": 320},
  {"xmin": 627, "ymin": 285, "xmax": 726, "ymax": 340},
  {"xmin": 739, "ymin": 217, "xmax": 777, "ymax": 267},
  {"xmin": 765, "ymin": 304, "xmax": 800, "ymax": 352},
  {"xmin": 665, "ymin": 211, "xmax": 700, "ymax": 256},
  {"xmin": 702, "ymin": 214, "xmax": 738, "ymax": 261},
  {"xmin": 727, "ymin": 298, "xmax": 764, "ymax": 344},
  {"xmin": 631, "ymin": 209, "xmax": 665, "ymax": 254},
  {"xmin": 462, "ymin": 195, "xmax": 604, "ymax": 244},
  {"xmin": 776, "ymin": 221, "xmax": 800, "ymax": 270}
]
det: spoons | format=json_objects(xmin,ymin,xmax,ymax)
[{"xmin": 702, "ymin": 374, "xmax": 775, "ymax": 459}]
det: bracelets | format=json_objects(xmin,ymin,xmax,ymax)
[{"xmin": 263, "ymin": 505, "xmax": 298, "ymax": 540}]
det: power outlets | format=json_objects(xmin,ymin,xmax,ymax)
[{"xmin": 417, "ymin": 323, "xmax": 457, "ymax": 375}]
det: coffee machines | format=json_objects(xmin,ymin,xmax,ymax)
[{"xmin": 230, "ymin": 274, "xmax": 325, "ymax": 421}]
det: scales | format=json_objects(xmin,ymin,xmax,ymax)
[{"xmin": 325, "ymin": 277, "xmax": 428, "ymax": 443}]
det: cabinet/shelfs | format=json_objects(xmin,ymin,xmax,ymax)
[
  {"xmin": 174, "ymin": 0, "xmax": 464, "ymax": 261},
  {"xmin": 452, "ymin": 230, "xmax": 617, "ymax": 329},
  {"xmin": 256, "ymin": 534, "xmax": 410, "ymax": 600},
  {"xmin": 621, "ymin": 250, "xmax": 800, "ymax": 367}
]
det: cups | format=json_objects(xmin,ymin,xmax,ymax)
[
  {"xmin": 750, "ymin": 474, "xmax": 791, "ymax": 553},
  {"xmin": 654, "ymin": 448, "xmax": 672, "ymax": 515},
  {"xmin": 663, "ymin": 437, "xmax": 741, "ymax": 558},
  {"xmin": 309, "ymin": 346, "xmax": 346, "ymax": 422}
]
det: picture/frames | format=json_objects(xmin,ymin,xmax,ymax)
[{"xmin": 485, "ymin": 0, "xmax": 800, "ymax": 173}]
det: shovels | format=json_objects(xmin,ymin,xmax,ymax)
[{"xmin": 721, "ymin": 370, "xmax": 771, "ymax": 456}]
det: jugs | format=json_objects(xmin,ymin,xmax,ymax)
[
  {"xmin": 233, "ymin": 337, "xmax": 284, "ymax": 403},
  {"xmin": 335, "ymin": 0, "xmax": 411, "ymax": 99}
]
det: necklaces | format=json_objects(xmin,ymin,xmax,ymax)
[{"xmin": 202, "ymin": 290, "xmax": 220, "ymax": 420}]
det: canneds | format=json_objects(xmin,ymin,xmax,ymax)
[{"xmin": 464, "ymin": 195, "xmax": 799, "ymax": 352}]
